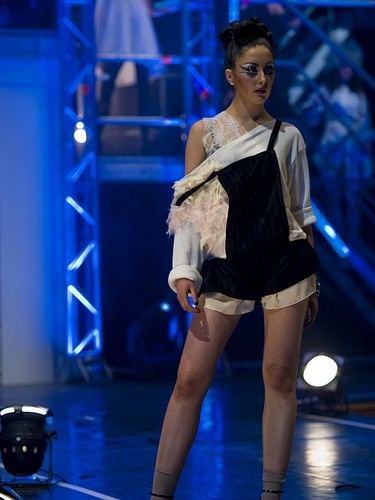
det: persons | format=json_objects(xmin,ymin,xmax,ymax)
[
  {"xmin": 300, "ymin": 39, "xmax": 375, "ymax": 249},
  {"xmin": 240, "ymin": 3, "xmax": 300, "ymax": 120},
  {"xmin": 288, "ymin": 6, "xmax": 374, "ymax": 121},
  {"xmin": 96, "ymin": 0, "xmax": 169, "ymax": 155},
  {"xmin": 151, "ymin": 15, "xmax": 321, "ymax": 500}
]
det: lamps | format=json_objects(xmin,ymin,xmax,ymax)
[
  {"xmin": 0, "ymin": 404, "xmax": 56, "ymax": 486},
  {"xmin": 296, "ymin": 351, "xmax": 350, "ymax": 416}
]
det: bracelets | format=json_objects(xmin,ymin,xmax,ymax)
[{"xmin": 315, "ymin": 282, "xmax": 321, "ymax": 294}]
